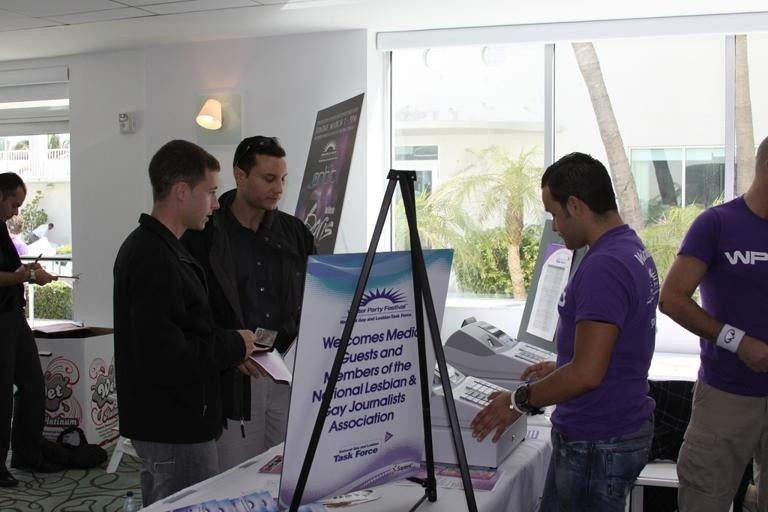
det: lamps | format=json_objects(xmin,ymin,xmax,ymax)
[{"xmin": 195, "ymin": 98, "xmax": 223, "ymax": 131}]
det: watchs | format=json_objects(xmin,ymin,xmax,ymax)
[{"xmin": 514, "ymin": 383, "xmax": 541, "ymax": 415}]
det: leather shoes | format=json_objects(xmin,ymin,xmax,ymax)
[
  {"xmin": 0, "ymin": 470, "xmax": 18, "ymax": 486},
  {"xmin": 11, "ymin": 454, "xmax": 59, "ymax": 472}
]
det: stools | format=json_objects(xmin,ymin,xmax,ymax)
[{"xmin": 630, "ymin": 458, "xmax": 735, "ymax": 512}]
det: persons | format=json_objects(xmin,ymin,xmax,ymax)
[
  {"xmin": 468, "ymin": 151, "xmax": 663, "ymax": 512},
  {"xmin": 655, "ymin": 133, "xmax": 768, "ymax": 512},
  {"xmin": 0, "ymin": 172, "xmax": 60, "ymax": 487},
  {"xmin": 112, "ymin": 136, "xmax": 264, "ymax": 502},
  {"xmin": 30, "ymin": 223, "xmax": 54, "ymax": 244},
  {"xmin": 6, "ymin": 212, "xmax": 30, "ymax": 295},
  {"xmin": 206, "ymin": 134, "xmax": 322, "ymax": 476}
]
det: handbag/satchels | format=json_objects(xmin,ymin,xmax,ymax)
[{"xmin": 40, "ymin": 426, "xmax": 107, "ymax": 468}]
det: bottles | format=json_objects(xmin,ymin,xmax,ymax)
[{"xmin": 123, "ymin": 491, "xmax": 136, "ymax": 512}]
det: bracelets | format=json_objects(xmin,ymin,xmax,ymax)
[
  {"xmin": 712, "ymin": 323, "xmax": 745, "ymax": 352},
  {"xmin": 511, "ymin": 390, "xmax": 527, "ymax": 417},
  {"xmin": 29, "ymin": 268, "xmax": 38, "ymax": 283}
]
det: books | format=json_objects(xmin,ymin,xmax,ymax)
[{"xmin": 249, "ymin": 345, "xmax": 294, "ymax": 388}]
